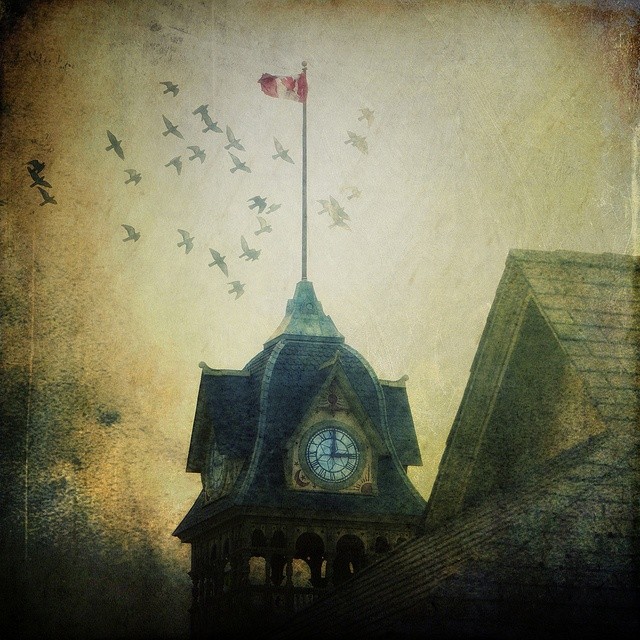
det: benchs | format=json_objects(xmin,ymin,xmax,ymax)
[{"xmin": 259, "ymin": 72, "xmax": 309, "ymax": 102}]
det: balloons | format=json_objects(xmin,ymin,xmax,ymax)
[
  {"xmin": 205, "ymin": 433, "xmax": 228, "ymax": 501},
  {"xmin": 299, "ymin": 421, "xmax": 366, "ymax": 492}
]
